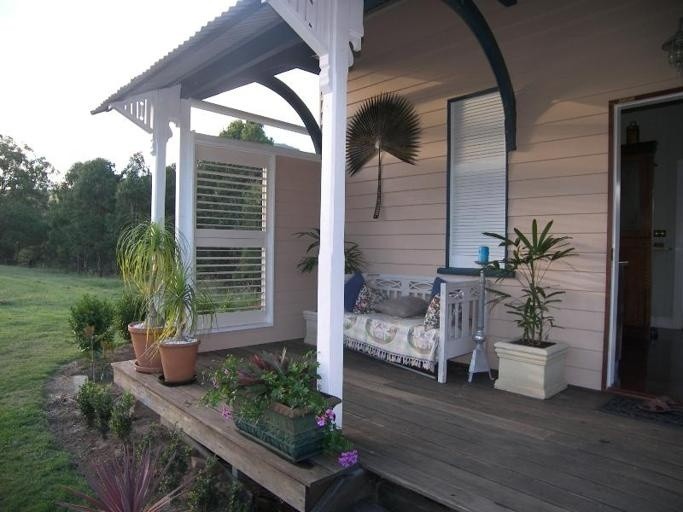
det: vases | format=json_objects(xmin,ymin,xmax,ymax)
[{"xmin": 230, "ymin": 382, "xmax": 340, "ymax": 464}]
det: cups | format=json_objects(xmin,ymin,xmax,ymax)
[{"xmin": 479, "ymin": 247, "xmax": 489, "ymax": 262}]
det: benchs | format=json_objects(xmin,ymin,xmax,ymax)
[{"xmin": 303, "ymin": 274, "xmax": 491, "ymax": 384}]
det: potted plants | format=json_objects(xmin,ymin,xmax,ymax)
[
  {"xmin": 483, "ymin": 217, "xmax": 576, "ymax": 400},
  {"xmin": 116, "ymin": 219, "xmax": 236, "ymax": 385}
]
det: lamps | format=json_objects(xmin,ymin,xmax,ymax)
[{"xmin": 662, "ymin": 27, "xmax": 683, "ymax": 70}]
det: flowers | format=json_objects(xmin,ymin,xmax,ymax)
[{"xmin": 196, "ymin": 351, "xmax": 362, "ymax": 466}]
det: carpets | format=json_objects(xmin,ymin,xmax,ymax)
[{"xmin": 594, "ymin": 393, "xmax": 683, "ymax": 428}]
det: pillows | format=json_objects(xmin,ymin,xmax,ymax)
[{"xmin": 344, "ymin": 272, "xmax": 464, "ymax": 330}]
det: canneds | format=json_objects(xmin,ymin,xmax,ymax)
[{"xmin": 478, "ymin": 246, "xmax": 489, "ymax": 263}]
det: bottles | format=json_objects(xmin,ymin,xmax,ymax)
[{"xmin": 626, "ymin": 121, "xmax": 639, "ymax": 144}]
